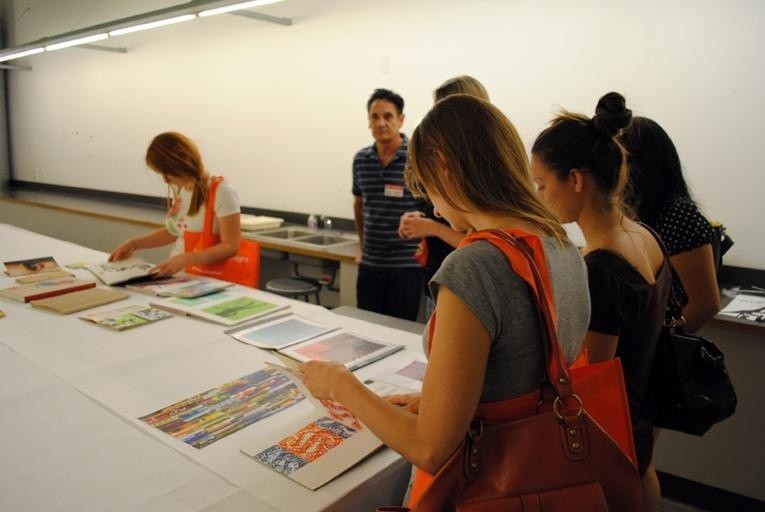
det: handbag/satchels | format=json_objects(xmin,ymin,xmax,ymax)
[
  {"xmin": 182, "ymin": 229, "xmax": 262, "ymax": 291},
  {"xmin": 475, "ymin": 346, "xmax": 640, "ymax": 471},
  {"xmin": 642, "ymin": 333, "xmax": 741, "ymax": 438},
  {"xmin": 407, "ymin": 405, "xmax": 641, "ymax": 510}
]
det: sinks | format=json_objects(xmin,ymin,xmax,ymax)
[
  {"xmin": 295, "ymin": 234, "xmax": 359, "ymax": 247},
  {"xmin": 258, "ymin": 229, "xmax": 314, "ymax": 239}
]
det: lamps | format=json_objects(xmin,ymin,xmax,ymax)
[{"xmin": 1, "ymin": 0, "xmax": 299, "ymax": 75}]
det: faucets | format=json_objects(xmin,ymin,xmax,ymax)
[{"xmin": 321, "ymin": 213, "xmax": 332, "ymax": 231}]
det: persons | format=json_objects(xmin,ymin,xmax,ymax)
[
  {"xmin": 107, "ymin": 132, "xmax": 241, "ymax": 280},
  {"xmin": 531, "ymin": 91, "xmax": 673, "ymax": 482},
  {"xmin": 613, "ymin": 117, "xmax": 721, "ymax": 512},
  {"xmin": 352, "ymin": 88, "xmax": 428, "ymax": 321},
  {"xmin": 299, "ymin": 94, "xmax": 592, "ymax": 512},
  {"xmin": 398, "ymin": 75, "xmax": 490, "ymax": 323}
]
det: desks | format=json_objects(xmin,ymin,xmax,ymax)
[{"xmin": 0, "ymin": 215, "xmax": 433, "ymax": 512}]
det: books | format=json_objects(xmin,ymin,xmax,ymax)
[
  {"xmin": 125, "ymin": 279, "xmax": 290, "ymax": 326},
  {"xmin": 80, "ymin": 304, "xmax": 173, "ymax": 332},
  {"xmin": 64, "ymin": 256, "xmax": 161, "ymax": 285},
  {"xmin": 1, "ymin": 256, "xmax": 129, "ymax": 316},
  {"xmin": 227, "ymin": 310, "xmax": 405, "ymax": 372},
  {"xmin": 242, "ymin": 348, "xmax": 418, "ymax": 491}
]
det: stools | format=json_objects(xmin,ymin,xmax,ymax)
[{"xmin": 266, "ymin": 274, "xmax": 322, "ymax": 305}]
